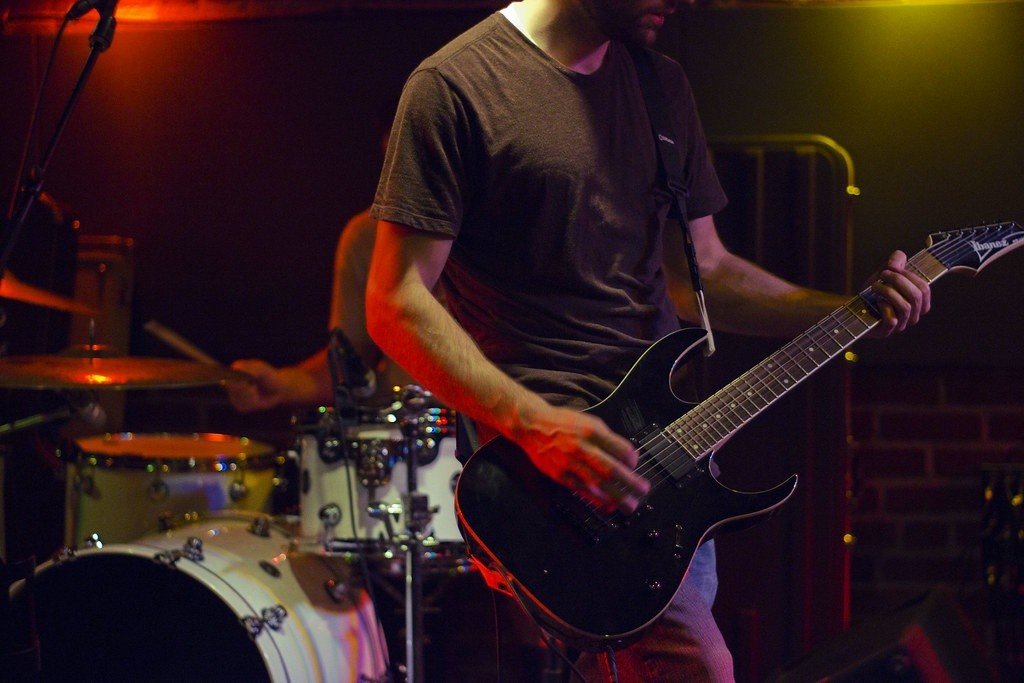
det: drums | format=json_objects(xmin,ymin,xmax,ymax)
[
  {"xmin": 288, "ymin": 401, "xmax": 477, "ymax": 572},
  {"xmin": 0, "ymin": 509, "xmax": 394, "ymax": 683},
  {"xmin": 55, "ymin": 428, "xmax": 292, "ymax": 554}
]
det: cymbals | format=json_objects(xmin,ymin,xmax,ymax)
[
  {"xmin": 0, "ymin": 344, "xmax": 259, "ymax": 394},
  {"xmin": 0, "ymin": 266, "xmax": 106, "ymax": 318}
]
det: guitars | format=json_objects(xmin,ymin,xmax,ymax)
[{"xmin": 454, "ymin": 219, "xmax": 1024, "ymax": 654}]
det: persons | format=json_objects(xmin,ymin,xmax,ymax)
[
  {"xmin": 222, "ymin": 106, "xmax": 414, "ymax": 418},
  {"xmin": 363, "ymin": 1, "xmax": 933, "ymax": 683}
]
